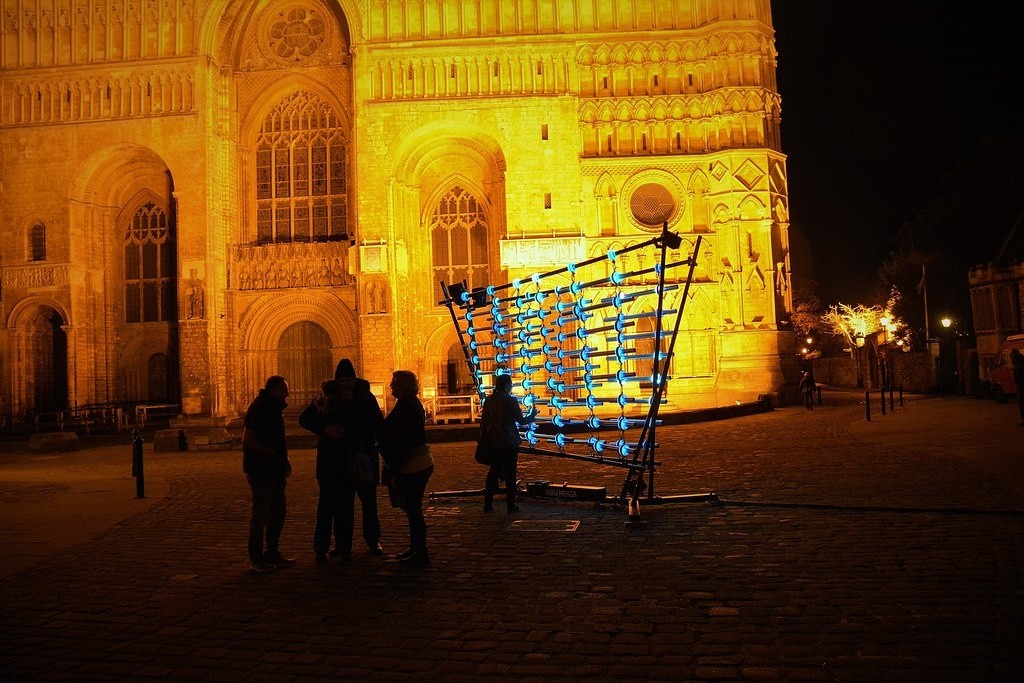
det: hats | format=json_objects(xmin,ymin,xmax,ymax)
[{"xmin": 335, "ymin": 359, "xmax": 356, "ymax": 379}]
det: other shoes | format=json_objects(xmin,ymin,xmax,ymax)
[
  {"xmin": 507, "ymin": 503, "xmax": 519, "ymax": 513},
  {"xmin": 484, "ymin": 500, "xmax": 493, "ymax": 512},
  {"xmin": 315, "ymin": 554, "xmax": 323, "ymax": 561},
  {"xmin": 249, "ymin": 559, "xmax": 296, "ymax": 573},
  {"xmin": 371, "ymin": 542, "xmax": 383, "ymax": 555},
  {"xmin": 1018, "ymin": 419, "xmax": 1024, "ymax": 426},
  {"xmin": 397, "ymin": 547, "xmax": 415, "ymax": 559},
  {"xmin": 400, "ymin": 552, "xmax": 427, "ymax": 565},
  {"xmin": 339, "ymin": 560, "xmax": 353, "ymax": 567}
]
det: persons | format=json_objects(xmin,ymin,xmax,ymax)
[
  {"xmin": 799, "ymin": 371, "xmax": 816, "ymax": 410},
  {"xmin": 300, "ymin": 358, "xmax": 385, "ymax": 563},
  {"xmin": 1010, "ymin": 349, "xmax": 1024, "ymax": 425},
  {"xmin": 478, "ymin": 374, "xmax": 536, "ymax": 513},
  {"xmin": 244, "ymin": 375, "xmax": 290, "ymax": 573},
  {"xmin": 381, "ymin": 371, "xmax": 433, "ymax": 567}
]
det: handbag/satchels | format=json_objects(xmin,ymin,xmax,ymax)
[{"xmin": 474, "ymin": 432, "xmax": 494, "ymax": 465}]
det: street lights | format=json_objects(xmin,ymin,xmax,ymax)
[
  {"xmin": 801, "ymin": 338, "xmax": 812, "ymax": 371},
  {"xmin": 881, "ymin": 314, "xmax": 891, "ymax": 390},
  {"xmin": 942, "ymin": 315, "xmax": 952, "ymax": 395}
]
